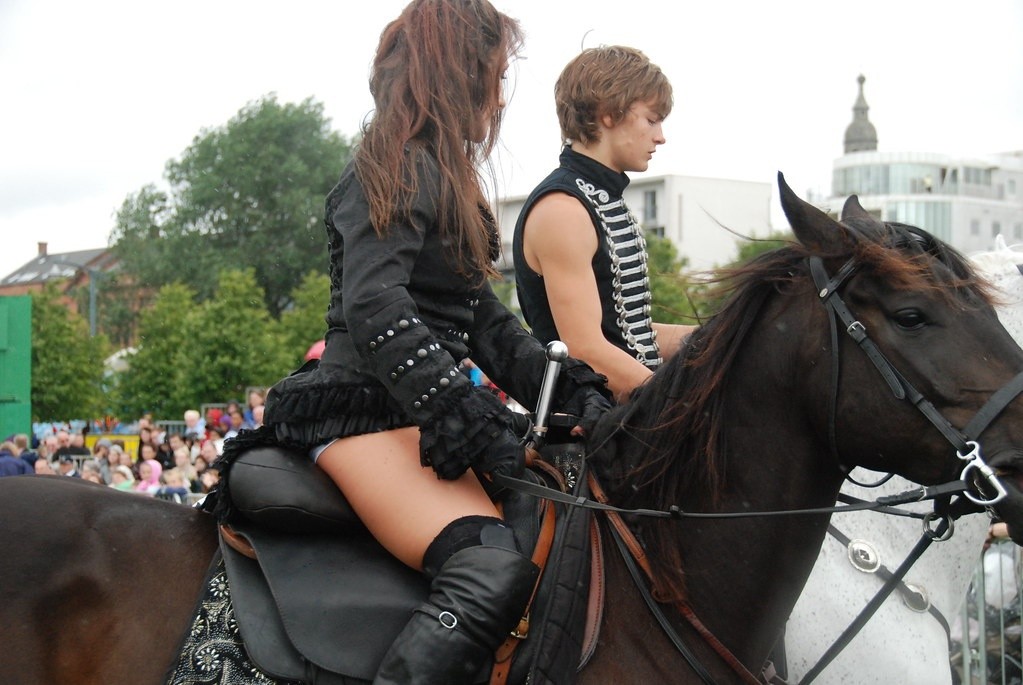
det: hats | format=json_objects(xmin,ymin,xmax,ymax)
[
  {"xmin": 59, "ymin": 455, "xmax": 73, "ymax": 464},
  {"xmin": 109, "ymin": 444, "xmax": 123, "ymax": 455},
  {"xmin": 97, "ymin": 438, "xmax": 111, "ymax": 450},
  {"xmin": 117, "ymin": 466, "xmax": 134, "ymax": 480}
]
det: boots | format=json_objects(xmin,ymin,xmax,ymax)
[{"xmin": 371, "ymin": 545, "xmax": 541, "ymax": 685}]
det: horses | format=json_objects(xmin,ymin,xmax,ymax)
[{"xmin": 0, "ymin": 169, "xmax": 1023, "ymax": 685}]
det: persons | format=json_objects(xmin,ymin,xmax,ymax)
[
  {"xmin": 0, "ymin": 385, "xmax": 273, "ymax": 508},
  {"xmin": 512, "ymin": 45, "xmax": 707, "ymax": 404},
  {"xmin": 262, "ymin": 0, "xmax": 617, "ymax": 685}
]
